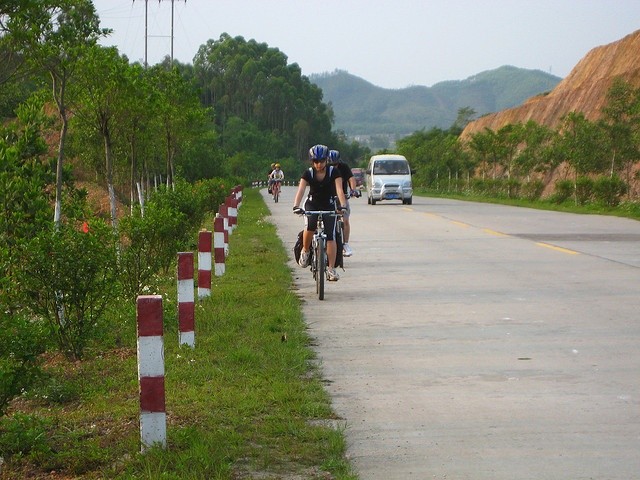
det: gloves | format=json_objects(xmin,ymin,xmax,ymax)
[{"xmin": 293, "ymin": 205, "xmax": 304, "ymax": 216}]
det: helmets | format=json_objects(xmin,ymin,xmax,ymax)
[
  {"xmin": 328, "ymin": 149, "xmax": 340, "ymax": 165},
  {"xmin": 270, "ymin": 163, "xmax": 275, "ymax": 168},
  {"xmin": 308, "ymin": 144, "xmax": 330, "ymax": 162},
  {"xmin": 275, "ymin": 164, "xmax": 281, "ymax": 168}
]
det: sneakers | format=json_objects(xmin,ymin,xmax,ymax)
[
  {"xmin": 325, "ymin": 265, "xmax": 340, "ymax": 281},
  {"xmin": 298, "ymin": 248, "xmax": 311, "ymax": 268},
  {"xmin": 341, "ymin": 242, "xmax": 353, "ymax": 257}
]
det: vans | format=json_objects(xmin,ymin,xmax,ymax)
[
  {"xmin": 367, "ymin": 154, "xmax": 413, "ymax": 205},
  {"xmin": 350, "ymin": 166, "xmax": 363, "ymax": 190}
]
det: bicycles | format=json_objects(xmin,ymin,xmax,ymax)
[
  {"xmin": 267, "ymin": 180, "xmax": 282, "ymax": 203},
  {"xmin": 293, "ymin": 207, "xmax": 340, "ymax": 300}
]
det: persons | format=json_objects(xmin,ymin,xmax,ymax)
[
  {"xmin": 267, "ymin": 163, "xmax": 276, "ymax": 191},
  {"xmin": 293, "ymin": 144, "xmax": 347, "ymax": 281},
  {"xmin": 269, "ymin": 164, "xmax": 284, "ymax": 192},
  {"xmin": 374, "ymin": 162, "xmax": 385, "ymax": 174},
  {"xmin": 328, "ymin": 150, "xmax": 359, "ymax": 256}
]
